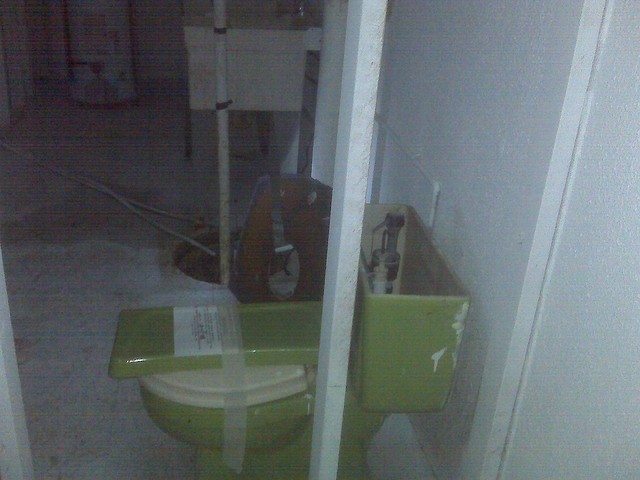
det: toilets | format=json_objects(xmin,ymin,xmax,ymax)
[{"xmin": 109, "ymin": 203, "xmax": 472, "ymax": 480}]
